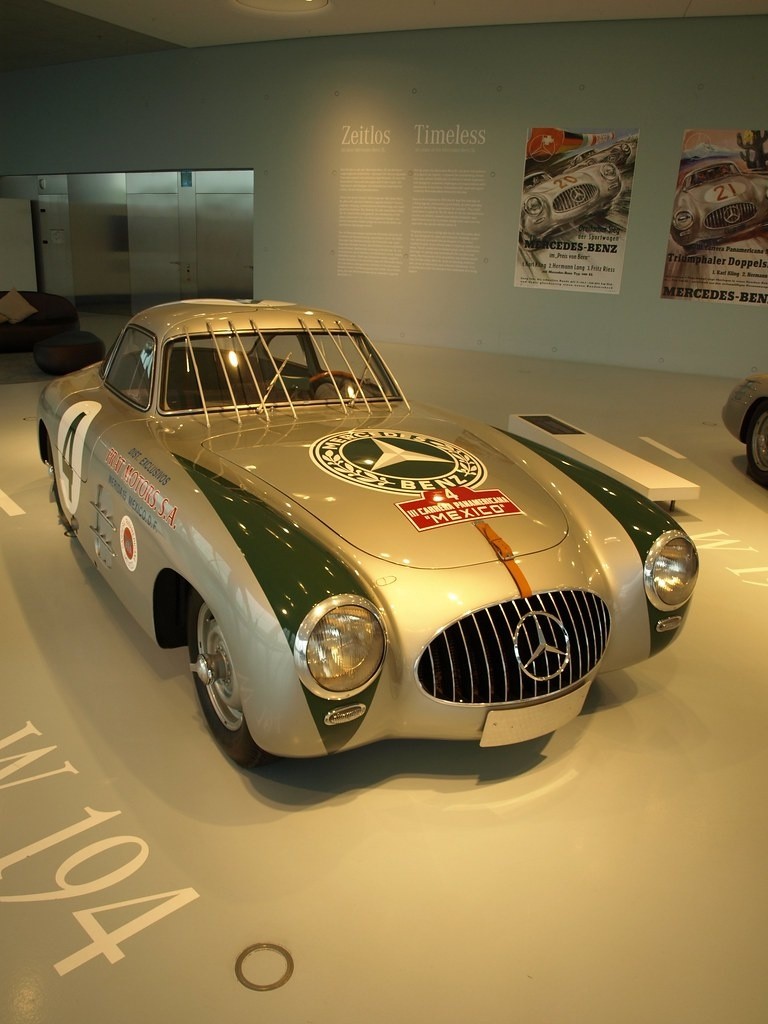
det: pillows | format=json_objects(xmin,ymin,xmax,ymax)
[
  {"xmin": 0, "ymin": 312, "xmax": 9, "ymax": 324},
  {"xmin": 0, "ymin": 286, "xmax": 40, "ymax": 326}
]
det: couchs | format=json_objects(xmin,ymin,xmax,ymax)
[{"xmin": 0, "ymin": 288, "xmax": 81, "ymax": 353}]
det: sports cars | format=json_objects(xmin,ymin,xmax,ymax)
[{"xmin": 38, "ymin": 299, "xmax": 699, "ymax": 768}]
